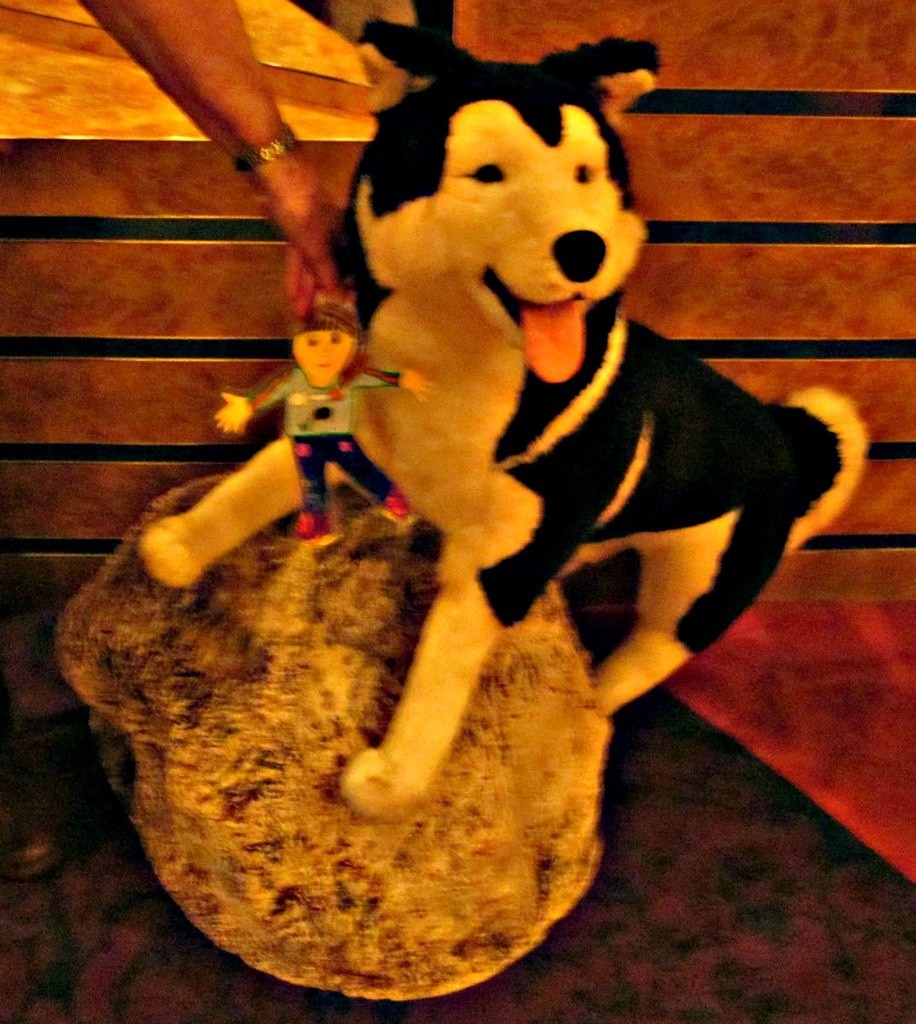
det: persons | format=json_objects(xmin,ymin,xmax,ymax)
[
  {"xmin": 75, "ymin": 0, "xmax": 344, "ymax": 317},
  {"xmin": 214, "ymin": 290, "xmax": 430, "ymax": 545}
]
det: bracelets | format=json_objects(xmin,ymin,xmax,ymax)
[{"xmin": 233, "ymin": 124, "xmax": 300, "ymax": 173}]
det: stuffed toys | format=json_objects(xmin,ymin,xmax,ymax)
[{"xmin": 136, "ymin": 16, "xmax": 869, "ymax": 821}]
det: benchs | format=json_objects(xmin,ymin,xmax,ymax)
[{"xmin": 0, "ymin": 1, "xmax": 916, "ymax": 1024}]
